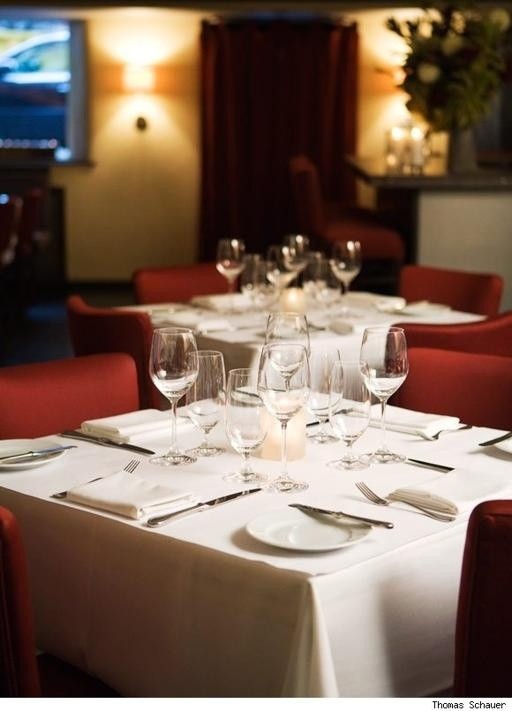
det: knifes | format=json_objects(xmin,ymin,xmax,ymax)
[
  {"xmin": 304, "ymin": 404, "xmax": 351, "ymax": 431},
  {"xmin": 1, "ymin": 444, "xmax": 79, "ymax": 464},
  {"xmin": 290, "ymin": 501, "xmax": 394, "ymax": 531},
  {"xmin": 60, "ymin": 428, "xmax": 155, "ymax": 458},
  {"xmin": 480, "ymin": 430, "xmax": 512, "ymax": 449},
  {"xmin": 147, "ymin": 486, "xmax": 261, "ymax": 528},
  {"xmin": 402, "ymin": 456, "xmax": 455, "ymax": 474}
]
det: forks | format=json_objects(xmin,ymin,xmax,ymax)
[
  {"xmin": 418, "ymin": 424, "xmax": 472, "ymax": 442},
  {"xmin": 54, "ymin": 457, "xmax": 144, "ymax": 503},
  {"xmin": 352, "ymin": 480, "xmax": 456, "ymax": 525}
]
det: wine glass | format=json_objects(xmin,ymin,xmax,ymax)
[
  {"xmin": 264, "ymin": 311, "xmax": 312, "ymax": 358},
  {"xmin": 257, "ymin": 343, "xmax": 311, "ymax": 497},
  {"xmin": 147, "ymin": 328, "xmax": 199, "ymax": 467},
  {"xmin": 357, "ymin": 326, "xmax": 411, "ymax": 464},
  {"xmin": 183, "ymin": 344, "xmax": 227, "ymax": 458},
  {"xmin": 324, "ymin": 361, "xmax": 374, "ymax": 475},
  {"xmin": 303, "ymin": 344, "xmax": 345, "ymax": 445},
  {"xmin": 214, "ymin": 233, "xmax": 364, "ymax": 323},
  {"xmin": 223, "ymin": 365, "xmax": 269, "ymax": 490}
]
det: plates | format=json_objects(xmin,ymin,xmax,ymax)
[
  {"xmin": 0, "ymin": 439, "xmax": 65, "ymax": 473},
  {"xmin": 244, "ymin": 509, "xmax": 371, "ymax": 552},
  {"xmin": 229, "ymin": 387, "xmax": 262, "ymax": 406}
]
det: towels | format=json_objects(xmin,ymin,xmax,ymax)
[
  {"xmin": 2, "ymin": 387, "xmax": 511, "ymax": 700},
  {"xmin": 65, "ymin": 468, "xmax": 194, "ymax": 520},
  {"xmin": 80, "ymin": 407, "xmax": 201, "ymax": 443},
  {"xmin": 386, "ymin": 451, "xmax": 511, "ymax": 517},
  {"xmin": 351, "ymin": 400, "xmax": 458, "ymax": 440}
]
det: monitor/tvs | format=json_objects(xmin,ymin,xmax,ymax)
[{"xmin": 0, "ymin": 9, "xmax": 88, "ymax": 169}]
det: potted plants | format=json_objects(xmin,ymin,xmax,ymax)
[{"xmin": 385, "ymin": 0, "xmax": 511, "ymax": 171}]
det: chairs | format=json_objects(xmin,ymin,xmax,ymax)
[
  {"xmin": 396, "ymin": 263, "xmax": 503, "ymax": 320},
  {"xmin": 384, "ymin": 311, "xmax": 512, "ymax": 360},
  {"xmin": 288, "ymin": 153, "xmax": 407, "ymax": 295},
  {"xmin": 65, "ymin": 295, "xmax": 186, "ymax": 411},
  {"xmin": 454, "ymin": 500, "xmax": 510, "ymax": 698},
  {"xmin": 133, "ymin": 263, "xmax": 249, "ymax": 304},
  {"xmin": 391, "ymin": 346, "xmax": 512, "ymax": 436},
  {"xmin": 2, "ymin": 354, "xmax": 141, "ymax": 440},
  {"xmin": 0, "ymin": 505, "xmax": 46, "ymax": 698}
]
